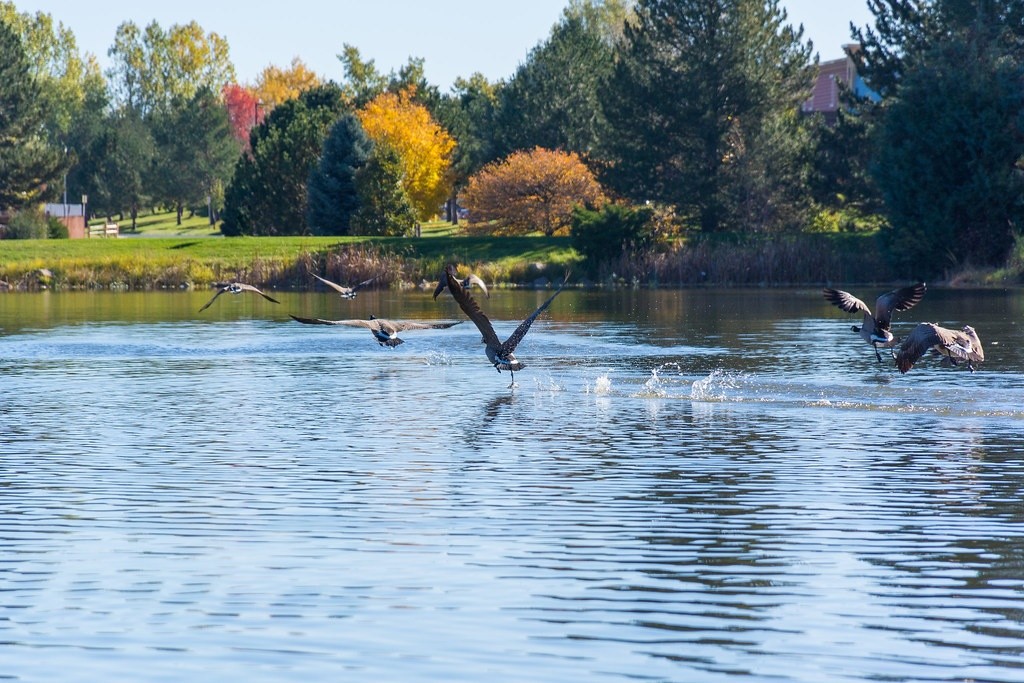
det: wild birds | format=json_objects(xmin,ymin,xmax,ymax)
[
  {"xmin": 822, "ymin": 280, "xmax": 985, "ymax": 374},
  {"xmin": 288, "ymin": 271, "xmax": 573, "ymax": 389},
  {"xmin": 198, "ymin": 283, "xmax": 280, "ymax": 313}
]
